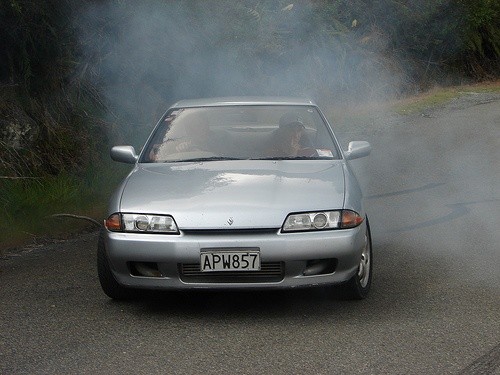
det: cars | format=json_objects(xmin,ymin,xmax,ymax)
[{"xmin": 96, "ymin": 97, "xmax": 374, "ymax": 300}]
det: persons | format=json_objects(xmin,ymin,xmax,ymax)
[
  {"xmin": 264, "ymin": 113, "xmax": 317, "ymax": 158},
  {"xmin": 172, "ymin": 111, "xmax": 225, "ymax": 155}
]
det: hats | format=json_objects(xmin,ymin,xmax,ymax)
[{"xmin": 279, "ymin": 112, "xmax": 306, "ymax": 129}]
